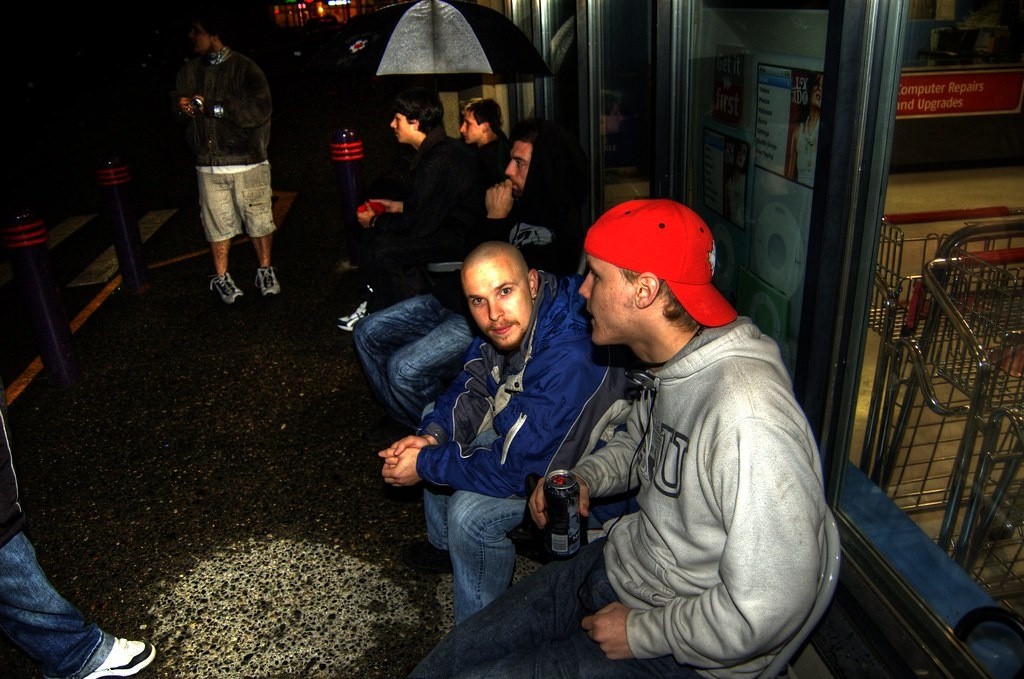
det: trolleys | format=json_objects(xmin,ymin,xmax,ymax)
[{"xmin": 861, "ymin": 207, "xmax": 1024, "ymax": 627}]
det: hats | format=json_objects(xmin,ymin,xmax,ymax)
[{"xmin": 584, "ymin": 199, "xmax": 738, "ymax": 327}]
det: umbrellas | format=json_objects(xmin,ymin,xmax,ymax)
[{"xmin": 308, "ymin": 1, "xmax": 552, "ymax": 80}]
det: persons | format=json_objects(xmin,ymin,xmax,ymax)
[
  {"xmin": 596, "ymin": 94, "xmax": 633, "ymax": 166},
  {"xmin": 333, "ymin": 86, "xmax": 843, "ymax": 679},
  {"xmin": 725, "ymin": 71, "xmax": 823, "ymax": 228},
  {"xmin": 0, "ymin": 393, "xmax": 156, "ymax": 678},
  {"xmin": 172, "ymin": 11, "xmax": 280, "ymax": 310}
]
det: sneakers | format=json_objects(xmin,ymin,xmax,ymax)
[
  {"xmin": 208, "ymin": 273, "xmax": 244, "ymax": 305},
  {"xmin": 255, "ymin": 266, "xmax": 282, "ymax": 300},
  {"xmin": 402, "ymin": 541, "xmax": 453, "ymax": 572},
  {"xmin": 337, "ymin": 302, "xmax": 372, "ymax": 332},
  {"xmin": 68, "ymin": 629, "xmax": 157, "ymax": 679}
]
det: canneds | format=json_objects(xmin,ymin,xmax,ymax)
[{"xmin": 543, "ymin": 470, "xmax": 581, "ymax": 559}]
